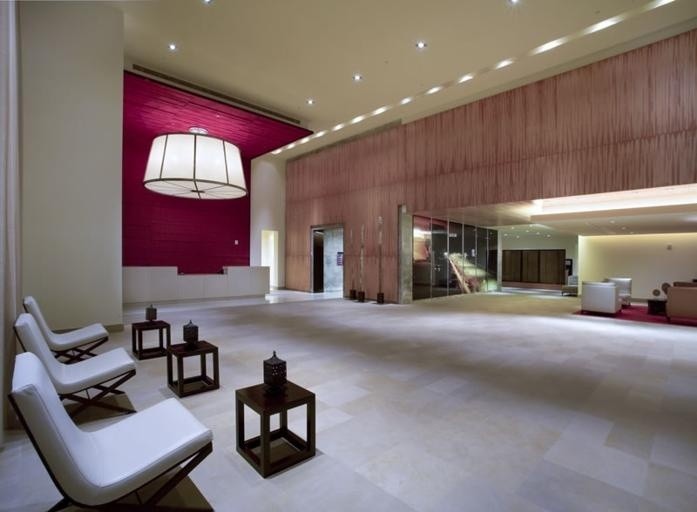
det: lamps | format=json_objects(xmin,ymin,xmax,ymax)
[{"xmin": 141, "ymin": 134, "xmax": 249, "ymax": 201}]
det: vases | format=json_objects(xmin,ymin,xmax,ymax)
[
  {"xmin": 349, "ymin": 288, "xmax": 365, "ymax": 302},
  {"xmin": 375, "ymin": 293, "xmax": 384, "ymax": 304}
]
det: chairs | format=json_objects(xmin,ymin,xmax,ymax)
[
  {"xmin": 19, "ymin": 295, "xmax": 111, "ymax": 364},
  {"xmin": 12, "ymin": 314, "xmax": 140, "ymax": 416},
  {"xmin": 11, "ymin": 351, "xmax": 213, "ymax": 511}
]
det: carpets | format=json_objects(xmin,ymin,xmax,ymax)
[{"xmin": 572, "ymin": 303, "xmax": 696, "ymax": 326}]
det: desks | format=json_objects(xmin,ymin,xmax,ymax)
[
  {"xmin": 235, "ymin": 379, "xmax": 314, "ymax": 478},
  {"xmin": 165, "ymin": 342, "xmax": 219, "ymax": 398},
  {"xmin": 131, "ymin": 319, "xmax": 172, "ymax": 360}
]
